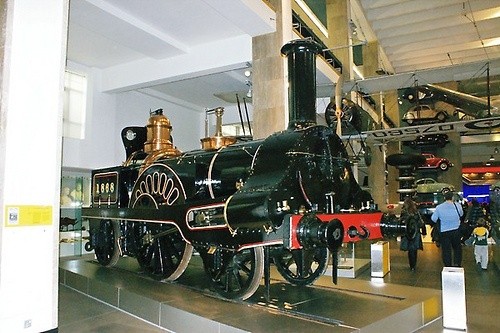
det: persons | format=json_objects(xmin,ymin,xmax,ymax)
[
  {"xmin": 400, "ymin": 198, "xmax": 427, "ymax": 270},
  {"xmin": 61, "ymin": 186, "xmax": 72, "ymax": 207},
  {"xmin": 471, "ymin": 217, "xmax": 490, "ymax": 271},
  {"xmin": 70, "ymin": 182, "xmax": 83, "ymax": 204},
  {"xmin": 431, "ymin": 190, "xmax": 465, "ymax": 269}
]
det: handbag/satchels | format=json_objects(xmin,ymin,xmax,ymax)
[
  {"xmin": 459, "ymin": 222, "xmax": 467, "ymax": 234},
  {"xmin": 487, "ymin": 235, "xmax": 497, "ymax": 244}
]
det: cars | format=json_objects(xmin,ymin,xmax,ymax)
[
  {"xmin": 413, "ymin": 179, "xmax": 456, "ymax": 197},
  {"xmin": 410, "ymin": 154, "xmax": 451, "ymax": 172},
  {"xmin": 416, "ymin": 203, "xmax": 436, "ymax": 216},
  {"xmin": 403, "ymin": 84, "xmax": 443, "ymax": 102},
  {"xmin": 401, "ymin": 104, "xmax": 451, "ymax": 124},
  {"xmin": 410, "ymin": 134, "xmax": 449, "ymax": 148}
]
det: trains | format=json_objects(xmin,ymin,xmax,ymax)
[{"xmin": 81, "ymin": 37, "xmax": 418, "ymax": 302}]
